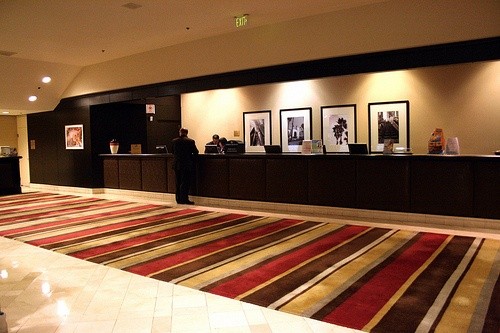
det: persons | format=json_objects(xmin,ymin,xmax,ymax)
[
  {"xmin": 206, "ymin": 134, "xmax": 227, "ymax": 154},
  {"xmin": 173, "ymin": 128, "xmax": 199, "ymax": 204}
]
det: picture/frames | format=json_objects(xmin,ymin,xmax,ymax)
[
  {"xmin": 65, "ymin": 124, "xmax": 84, "ymax": 150},
  {"xmin": 320, "ymin": 103, "xmax": 357, "ymax": 152},
  {"xmin": 242, "ymin": 110, "xmax": 272, "ymax": 152},
  {"xmin": 279, "ymin": 106, "xmax": 313, "ymax": 152},
  {"xmin": 366, "ymin": 99, "xmax": 411, "ymax": 154}
]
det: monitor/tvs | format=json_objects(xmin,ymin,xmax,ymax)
[
  {"xmin": 205, "ymin": 144, "xmax": 220, "ymax": 153},
  {"xmin": 347, "ymin": 143, "xmax": 369, "ymax": 154},
  {"xmin": 225, "ymin": 143, "xmax": 240, "ymax": 152},
  {"xmin": 264, "ymin": 145, "xmax": 282, "ymax": 153}
]
